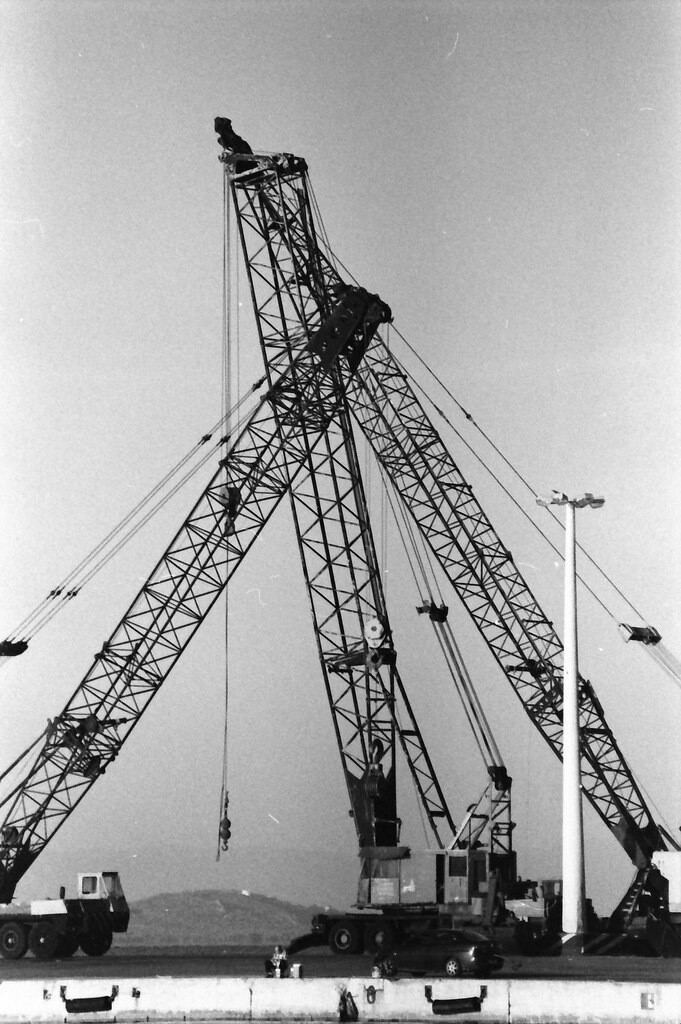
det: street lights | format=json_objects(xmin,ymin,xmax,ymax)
[{"xmin": 534, "ymin": 487, "xmax": 606, "ymax": 958}]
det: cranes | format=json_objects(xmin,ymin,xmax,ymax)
[{"xmin": 1, "ymin": 116, "xmax": 681, "ymax": 961}]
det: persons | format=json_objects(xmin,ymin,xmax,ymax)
[{"xmin": 266, "ymin": 944, "xmax": 289, "ymax": 978}]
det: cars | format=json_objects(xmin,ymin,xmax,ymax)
[{"xmin": 371, "ymin": 929, "xmax": 505, "ymax": 978}]
